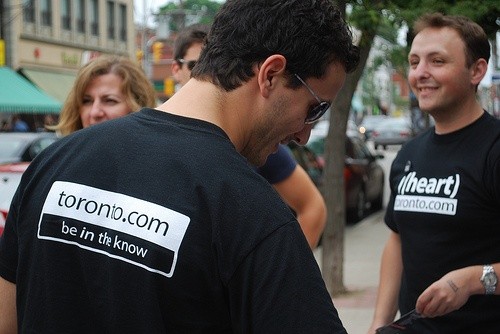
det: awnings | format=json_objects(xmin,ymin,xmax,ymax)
[
  {"xmin": 0, "ymin": 66, "xmax": 63, "ymax": 113},
  {"xmin": 21, "ymin": 69, "xmax": 76, "ymax": 102}
]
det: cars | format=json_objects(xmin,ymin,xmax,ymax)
[
  {"xmin": 362, "ymin": 114, "xmax": 411, "ymax": 149},
  {"xmin": 0, "ymin": 131, "xmax": 61, "ymax": 166},
  {"xmin": 289, "ymin": 129, "xmax": 386, "ymax": 222}
]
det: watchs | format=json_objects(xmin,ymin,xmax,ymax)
[{"xmin": 480, "ymin": 265, "xmax": 498, "ymax": 295}]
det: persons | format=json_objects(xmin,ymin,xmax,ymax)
[
  {"xmin": 45, "ymin": 58, "xmax": 157, "ymax": 138},
  {"xmin": 1, "ymin": 0, "xmax": 360, "ymax": 334},
  {"xmin": 13, "ymin": 113, "xmax": 56, "ymax": 131},
  {"xmin": 171, "ymin": 23, "xmax": 327, "ymax": 254},
  {"xmin": 365, "ymin": 12, "xmax": 500, "ymax": 334}
]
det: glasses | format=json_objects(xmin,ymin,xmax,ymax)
[
  {"xmin": 176, "ymin": 57, "xmax": 197, "ymax": 70},
  {"xmin": 294, "ymin": 72, "xmax": 331, "ymax": 125}
]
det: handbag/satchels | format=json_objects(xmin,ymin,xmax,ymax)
[{"xmin": 375, "ymin": 291, "xmax": 500, "ymax": 334}]
